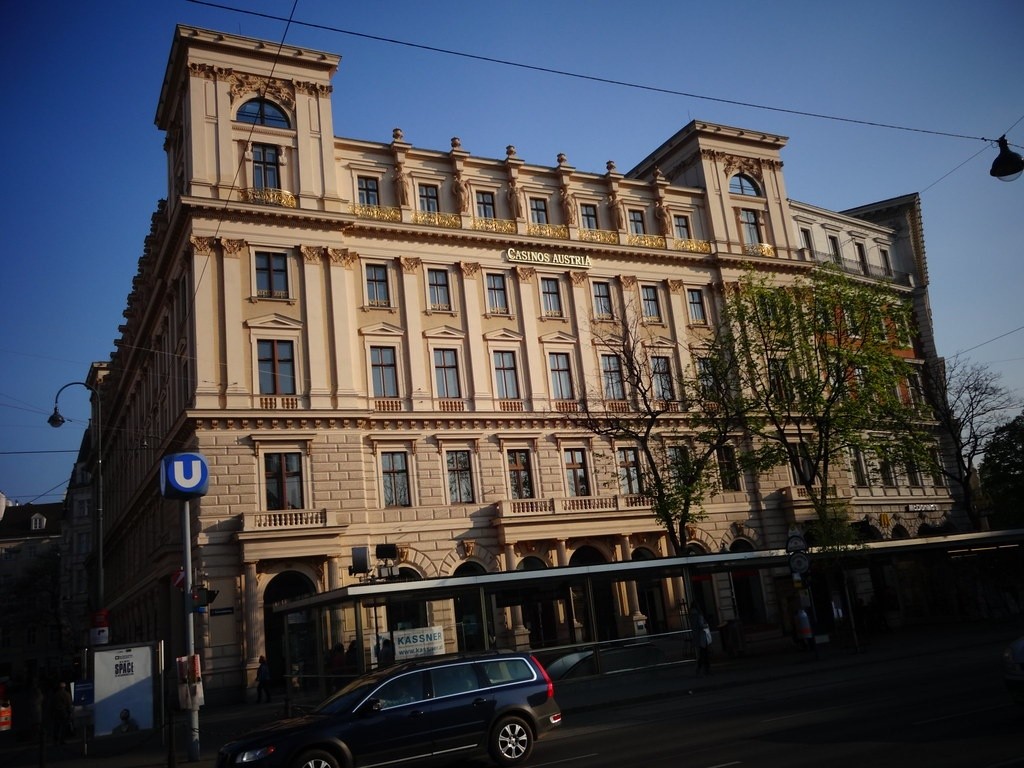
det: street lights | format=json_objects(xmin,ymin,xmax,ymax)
[{"xmin": 50, "ymin": 382, "xmax": 106, "ymax": 651}]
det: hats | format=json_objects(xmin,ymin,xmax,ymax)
[{"xmin": 331, "ymin": 643, "xmax": 343, "ymax": 656}]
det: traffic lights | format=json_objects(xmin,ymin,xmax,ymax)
[{"xmin": 197, "ymin": 588, "xmax": 219, "ymax": 608}]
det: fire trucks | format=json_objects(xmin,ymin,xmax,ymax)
[{"xmin": 0, "ymin": 677, "xmax": 13, "ymax": 732}]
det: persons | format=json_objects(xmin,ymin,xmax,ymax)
[
  {"xmin": 373, "ymin": 678, "xmax": 416, "ymax": 709},
  {"xmin": 255, "ymin": 655, "xmax": 272, "ymax": 704},
  {"xmin": 51, "ymin": 682, "xmax": 72, "ymax": 747},
  {"xmin": 111, "ymin": 709, "xmax": 139, "ymax": 735},
  {"xmin": 328, "ymin": 640, "xmax": 362, "ymax": 692},
  {"xmin": 689, "ymin": 601, "xmax": 714, "ymax": 678},
  {"xmin": 376, "ymin": 639, "xmax": 395, "ymax": 668}
]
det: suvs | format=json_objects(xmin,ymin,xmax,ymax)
[{"xmin": 214, "ymin": 649, "xmax": 563, "ymax": 768}]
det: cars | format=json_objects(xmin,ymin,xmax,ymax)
[{"xmin": 1002, "ymin": 635, "xmax": 1024, "ymax": 682}]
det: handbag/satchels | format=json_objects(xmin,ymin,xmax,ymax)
[{"xmin": 700, "ymin": 626, "xmax": 712, "ymax": 649}]
